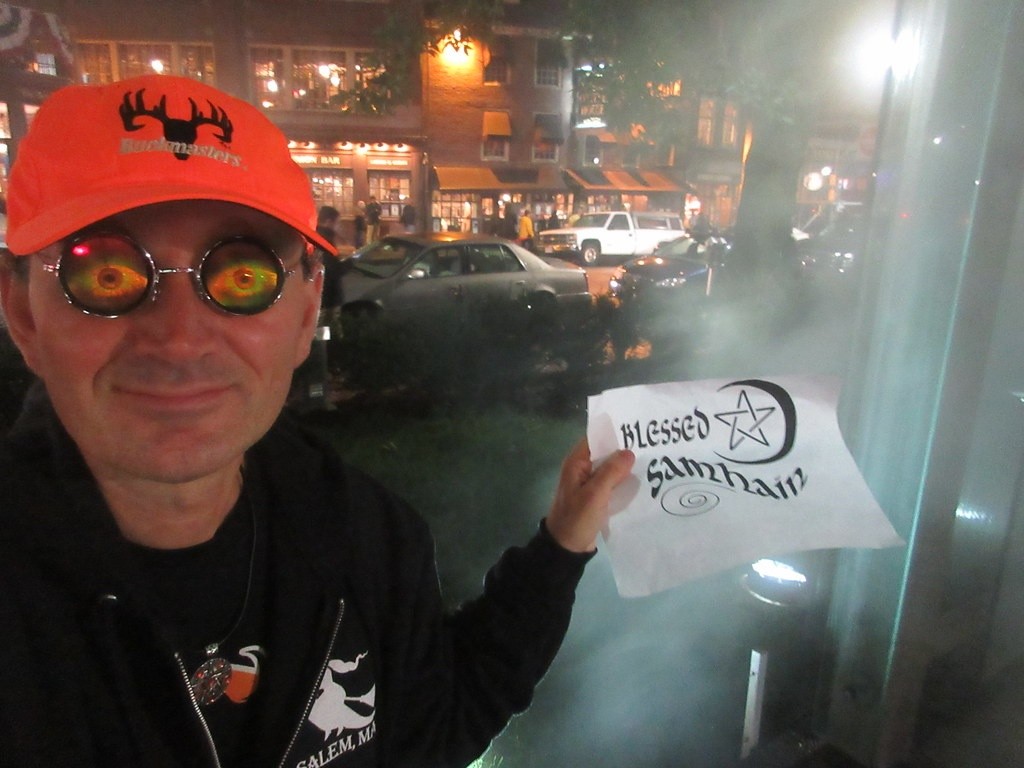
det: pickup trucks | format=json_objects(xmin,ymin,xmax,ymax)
[{"xmin": 535, "ymin": 211, "xmax": 686, "ymax": 265}]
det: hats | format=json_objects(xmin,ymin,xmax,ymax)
[{"xmin": 6, "ymin": 77, "xmax": 341, "ymax": 258}]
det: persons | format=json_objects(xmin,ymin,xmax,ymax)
[
  {"xmin": 400, "ymin": 197, "xmax": 417, "ymax": 232},
  {"xmin": 568, "ymin": 208, "xmax": 582, "ymax": 226},
  {"xmin": 489, "ymin": 205, "xmax": 535, "ymax": 251},
  {"xmin": 548, "ymin": 211, "xmax": 559, "ymax": 229},
  {"xmin": 0, "ymin": 74, "xmax": 636, "ymax": 768},
  {"xmin": 536, "ymin": 212, "xmax": 546, "ymax": 230},
  {"xmin": 353, "ymin": 196, "xmax": 381, "ymax": 249},
  {"xmin": 315, "ymin": 205, "xmax": 353, "ymax": 376},
  {"xmin": 689, "ymin": 209, "xmax": 710, "ymax": 231}
]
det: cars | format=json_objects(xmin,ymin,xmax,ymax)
[
  {"xmin": 323, "ymin": 235, "xmax": 591, "ymax": 349},
  {"xmin": 609, "ymin": 228, "xmax": 809, "ymax": 304}
]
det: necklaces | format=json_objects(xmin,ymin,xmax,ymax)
[{"xmin": 190, "ymin": 464, "xmax": 257, "ymax": 706}]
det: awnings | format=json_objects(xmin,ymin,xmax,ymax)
[
  {"xmin": 435, "ymin": 167, "xmax": 567, "ymax": 191},
  {"xmin": 534, "ymin": 115, "xmax": 564, "ymax": 139},
  {"xmin": 565, "ymin": 167, "xmax": 683, "ymax": 192},
  {"xmin": 482, "ymin": 111, "xmax": 512, "ymax": 138}
]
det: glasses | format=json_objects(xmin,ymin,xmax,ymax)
[{"xmin": 34, "ymin": 227, "xmax": 303, "ymax": 318}]
